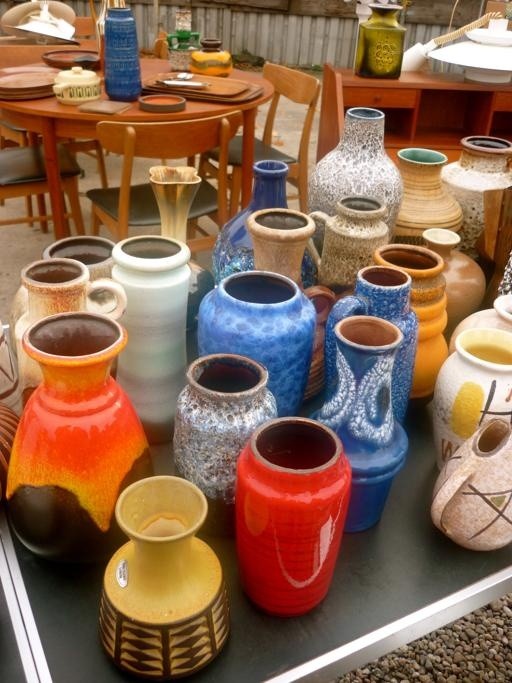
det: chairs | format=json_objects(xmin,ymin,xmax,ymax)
[
  {"xmin": 73, "ymin": 14, "xmax": 101, "ymax": 46},
  {"xmin": 195, "ymin": 61, "xmax": 322, "ymax": 218}
]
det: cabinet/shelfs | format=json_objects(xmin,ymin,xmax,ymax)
[{"xmin": 315, "ymin": 60, "xmax": 512, "ymax": 162}]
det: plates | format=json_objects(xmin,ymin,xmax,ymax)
[
  {"xmin": 40, "ymin": 49, "xmax": 100, "ymax": 69},
  {"xmin": 138, "ymin": 94, "xmax": 185, "ymax": 113},
  {"xmin": 0, "ymin": 1, "xmax": 76, "ymax": 39},
  {"xmin": 1, "ymin": 67, "xmax": 63, "ymax": 100}
]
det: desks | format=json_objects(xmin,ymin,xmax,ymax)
[
  {"xmin": 1, "ymin": 33, "xmax": 109, "ymax": 227},
  {"xmin": 1, "ymin": 54, "xmax": 278, "ymax": 242}
]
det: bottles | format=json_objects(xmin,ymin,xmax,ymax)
[{"xmin": 103, "ymin": 7, "xmax": 142, "ymax": 100}]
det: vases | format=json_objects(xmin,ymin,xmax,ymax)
[
  {"xmin": 2, "ymin": 310, "xmax": 160, "ymax": 573},
  {"xmin": 427, "ymin": 416, "xmax": 511, "ymax": 553},
  {"xmin": 2, "ymin": 402, "xmax": 511, "ymax": 683},
  {"xmin": 96, "ymin": 474, "xmax": 233, "ymax": 682},
  {"xmin": 352, "ymin": 1, "xmax": 408, "ymax": 80},
  {"xmin": 231, "ymin": 414, "xmax": 354, "ymax": 617},
  {"xmin": 188, "ymin": 36, "xmax": 234, "ymax": 77}
]
